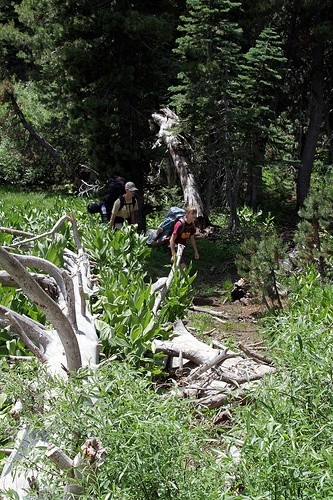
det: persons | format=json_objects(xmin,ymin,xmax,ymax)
[
  {"xmin": 103, "ymin": 171, "xmax": 139, "ymax": 231},
  {"xmin": 169, "ymin": 205, "xmax": 199, "ymax": 271}
]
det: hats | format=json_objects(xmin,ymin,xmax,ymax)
[{"xmin": 125, "ymin": 181, "xmax": 137, "ymax": 191}]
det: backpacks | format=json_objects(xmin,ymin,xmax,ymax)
[
  {"xmin": 154, "ymin": 206, "xmax": 187, "ymax": 240},
  {"xmin": 104, "ymin": 176, "xmax": 127, "ymax": 211}
]
